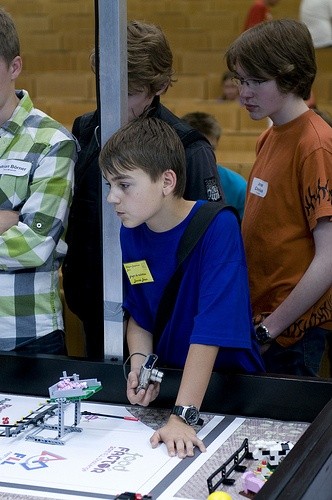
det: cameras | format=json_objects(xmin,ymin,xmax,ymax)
[{"xmin": 135, "ymin": 354, "xmax": 164, "ymax": 393}]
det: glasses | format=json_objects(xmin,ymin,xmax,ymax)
[{"xmin": 231, "ymin": 77, "xmax": 270, "ymax": 88}]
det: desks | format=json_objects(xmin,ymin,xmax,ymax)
[{"xmin": 0, "ymin": 350, "xmax": 332, "ymax": 500}]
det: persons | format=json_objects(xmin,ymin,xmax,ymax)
[
  {"xmin": 0, "ymin": 8, "xmax": 79, "ymax": 356},
  {"xmin": 99, "ymin": 119, "xmax": 268, "ymax": 459},
  {"xmin": 220, "ymin": 0, "xmax": 332, "ymax": 374},
  {"xmin": 180, "ymin": 112, "xmax": 249, "ymax": 220},
  {"xmin": 60, "ymin": 19, "xmax": 225, "ymax": 365}
]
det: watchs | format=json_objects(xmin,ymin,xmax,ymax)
[
  {"xmin": 168, "ymin": 404, "xmax": 204, "ymax": 427},
  {"xmin": 254, "ymin": 322, "xmax": 275, "ymax": 346}
]
